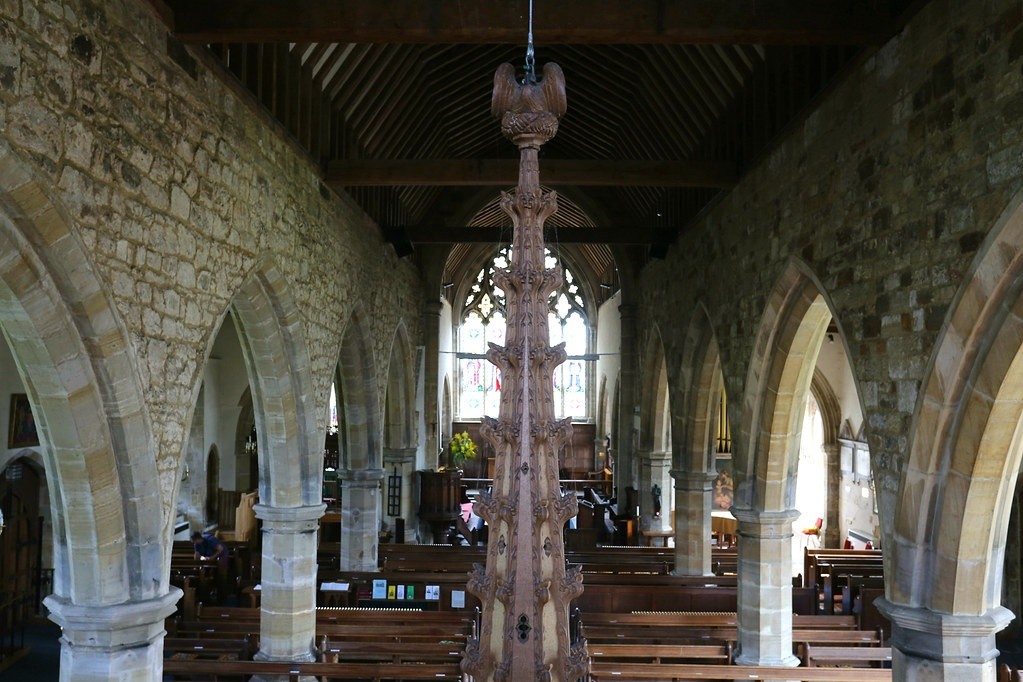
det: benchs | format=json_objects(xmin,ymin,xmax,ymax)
[{"xmin": 162, "ymin": 466, "xmax": 892, "ymax": 682}]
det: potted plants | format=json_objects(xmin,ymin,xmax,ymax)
[{"xmin": 440, "ymin": 430, "xmax": 478, "ymax": 503}]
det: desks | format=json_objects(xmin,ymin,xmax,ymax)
[{"xmin": 711, "ymin": 511, "xmax": 738, "ymax": 547}]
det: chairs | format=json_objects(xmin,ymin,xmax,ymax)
[
  {"xmin": 865, "ymin": 541, "xmax": 875, "ymax": 550},
  {"xmin": 844, "ymin": 537, "xmax": 854, "ymax": 549},
  {"xmin": 800, "ymin": 518, "xmax": 822, "ymax": 548}
]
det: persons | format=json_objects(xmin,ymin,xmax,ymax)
[{"xmin": 191, "ymin": 531, "xmax": 228, "ymax": 580}]
